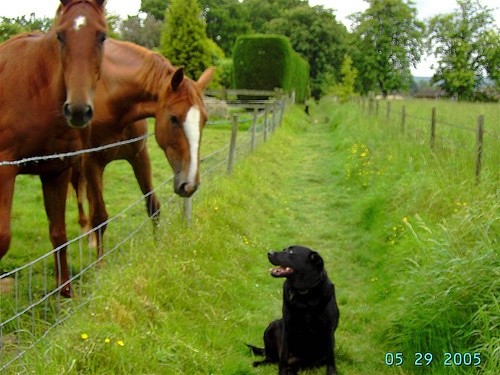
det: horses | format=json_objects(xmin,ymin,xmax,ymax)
[
  {"xmin": 68, "ymin": 36, "xmax": 216, "ymax": 271},
  {"xmin": 0, "ymin": 0, "xmax": 107, "ymax": 298}
]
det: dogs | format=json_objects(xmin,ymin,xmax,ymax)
[{"xmin": 244, "ymin": 246, "xmax": 339, "ymax": 375}]
